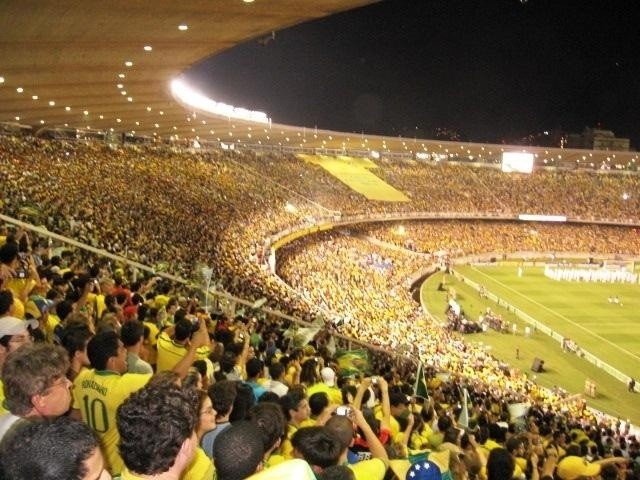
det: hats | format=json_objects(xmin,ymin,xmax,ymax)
[
  {"xmin": 0, "ymin": 316, "xmax": 40, "ymax": 338},
  {"xmin": 321, "ymin": 367, "xmax": 335, "ymax": 387}
]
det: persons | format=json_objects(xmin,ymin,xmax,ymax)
[{"xmin": 1, "ymin": 129, "xmax": 639, "ymax": 480}]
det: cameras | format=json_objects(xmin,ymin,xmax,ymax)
[
  {"xmin": 335, "ymin": 406, "xmax": 351, "ymax": 417},
  {"xmin": 371, "ymin": 376, "xmax": 381, "ymax": 384},
  {"xmin": 17, "ymin": 270, "xmax": 29, "ymax": 279},
  {"xmin": 192, "ymin": 315, "xmax": 200, "ymax": 323},
  {"xmin": 239, "ymin": 333, "xmax": 245, "ymax": 340}
]
